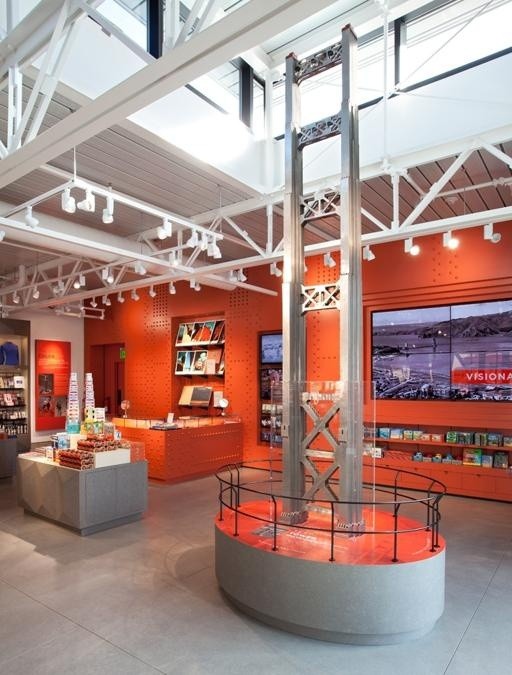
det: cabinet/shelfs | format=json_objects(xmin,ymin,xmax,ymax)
[
  {"xmin": 363, "ymin": 420, "xmax": 512, "ymax": 504},
  {"xmin": 170, "ymin": 310, "xmax": 225, "ymax": 414},
  {"xmin": 257, "ymin": 329, "xmax": 283, "ymax": 444},
  {"xmin": 1, "ymin": 319, "xmax": 31, "ymax": 479}
]
